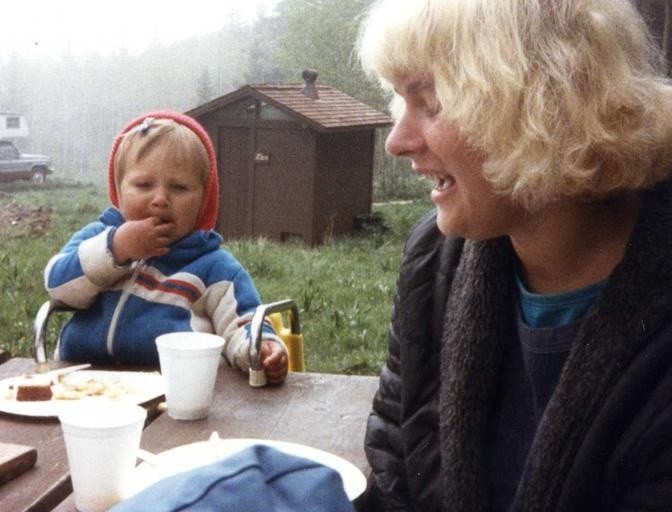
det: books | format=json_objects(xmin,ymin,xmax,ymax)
[{"xmin": 0, "ymin": 440, "xmax": 38, "ymax": 484}]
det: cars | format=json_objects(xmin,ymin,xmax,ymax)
[{"xmin": 0, "ymin": 139, "xmax": 55, "ymax": 186}]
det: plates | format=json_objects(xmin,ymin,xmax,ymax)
[
  {"xmin": 1, "ymin": 371, "xmax": 164, "ymax": 422},
  {"xmin": 132, "ymin": 435, "xmax": 367, "ymax": 502}
]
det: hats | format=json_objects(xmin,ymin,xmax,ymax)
[{"xmin": 108, "ymin": 110, "xmax": 219, "ymax": 229}]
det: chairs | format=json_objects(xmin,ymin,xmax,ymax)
[{"xmin": 34, "ymin": 297, "xmax": 305, "ymax": 386}]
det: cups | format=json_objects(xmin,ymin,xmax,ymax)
[
  {"xmin": 59, "ymin": 396, "xmax": 147, "ymax": 510},
  {"xmin": 155, "ymin": 329, "xmax": 226, "ymax": 421}
]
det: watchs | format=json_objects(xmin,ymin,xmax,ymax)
[{"xmin": 350, "ymin": 0, "xmax": 671, "ymax": 512}]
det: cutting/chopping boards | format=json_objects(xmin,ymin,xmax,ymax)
[{"xmin": 0, "ymin": 440, "xmax": 40, "ymax": 489}]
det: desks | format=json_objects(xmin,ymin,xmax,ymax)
[{"xmin": 0, "ymin": 356, "xmax": 380, "ymax": 511}]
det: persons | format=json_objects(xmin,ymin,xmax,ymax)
[{"xmin": 41, "ymin": 109, "xmax": 290, "ymax": 386}]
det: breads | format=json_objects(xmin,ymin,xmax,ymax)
[{"xmin": 16, "ymin": 384, "xmax": 52, "ymax": 401}]
